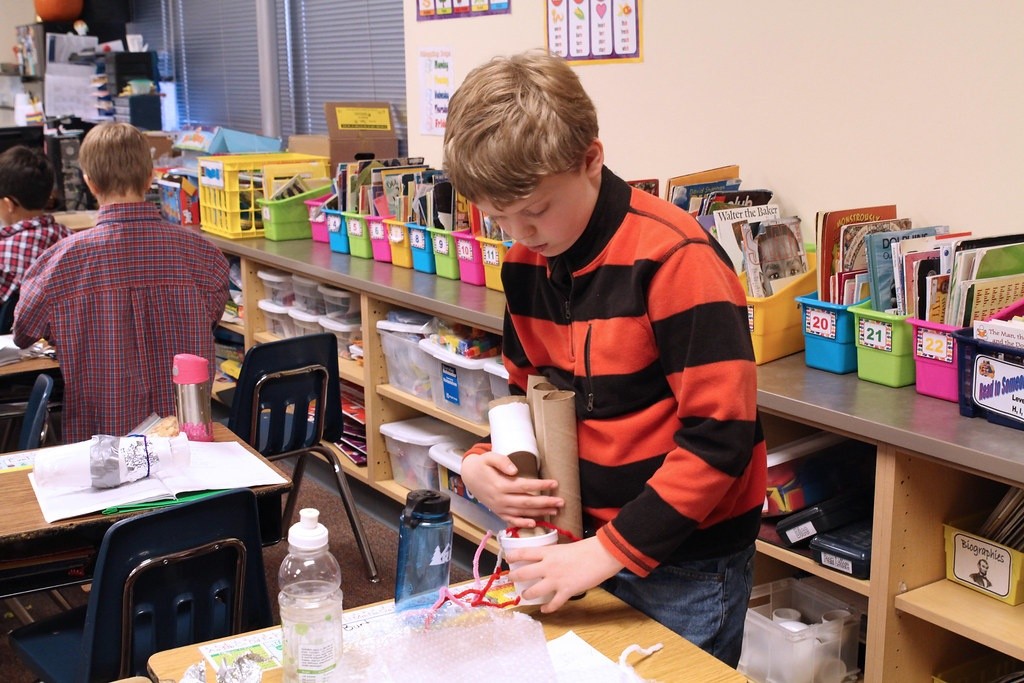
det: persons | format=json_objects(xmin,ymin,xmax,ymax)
[
  {"xmin": 758, "ymin": 224, "xmax": 806, "ymax": 298},
  {"xmin": 13, "ymin": 122, "xmax": 230, "ymax": 446},
  {"xmin": 444, "ymin": 50, "xmax": 767, "ymax": 670},
  {"xmin": 0, "ymin": 145, "xmax": 76, "ymax": 301}
]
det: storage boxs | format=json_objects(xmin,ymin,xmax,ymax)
[
  {"xmin": 144, "ymin": 101, "xmax": 1024, "ymax": 683},
  {"xmin": 106, "ymin": 47, "xmax": 161, "ymax": 131}
]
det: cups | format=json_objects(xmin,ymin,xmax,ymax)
[
  {"xmin": 772, "ymin": 606, "xmax": 853, "ymax": 683},
  {"xmin": 126, "ymin": 34, "xmax": 143, "ymax": 53},
  {"xmin": 498, "ymin": 529, "xmax": 559, "ymax": 606}
]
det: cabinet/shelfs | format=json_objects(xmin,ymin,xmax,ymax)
[{"xmin": 177, "ymin": 219, "xmax": 1024, "ymax": 682}]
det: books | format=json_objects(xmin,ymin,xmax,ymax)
[
  {"xmin": 334, "ymin": 157, "xmax": 511, "ymax": 242},
  {"xmin": 666, "ymin": 166, "xmax": 1024, "ymax": 327}
]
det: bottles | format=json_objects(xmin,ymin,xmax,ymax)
[
  {"xmin": 277, "ymin": 508, "xmax": 342, "ymax": 683},
  {"xmin": 172, "ymin": 354, "xmax": 214, "ymax": 443},
  {"xmin": 396, "ymin": 490, "xmax": 454, "ymax": 612}
]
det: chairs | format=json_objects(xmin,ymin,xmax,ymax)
[{"xmin": 0, "ymin": 332, "xmax": 378, "ymax": 683}]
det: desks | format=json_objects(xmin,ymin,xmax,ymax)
[
  {"xmin": 45, "ymin": 209, "xmax": 99, "ymax": 231},
  {"xmin": 0, "ymin": 421, "xmax": 295, "ymax": 600},
  {"xmin": 0, "ymin": 338, "xmax": 79, "ymax": 452},
  {"xmin": 145, "ymin": 556, "xmax": 748, "ymax": 683}
]
